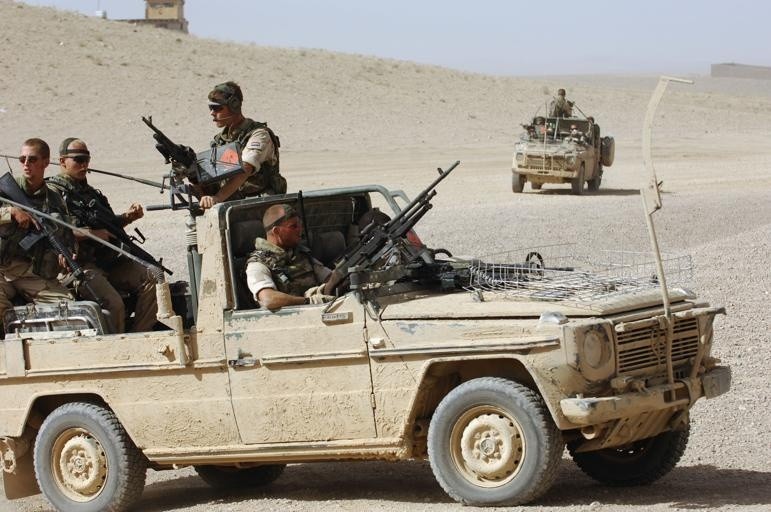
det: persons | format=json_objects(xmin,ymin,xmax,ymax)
[
  {"xmin": 46, "ymin": 135, "xmax": 159, "ymax": 335},
  {"xmin": 525, "ymin": 115, "xmax": 611, "ymax": 149},
  {"xmin": 548, "ymin": 87, "xmax": 574, "ymax": 118},
  {"xmin": 244, "ymin": 203, "xmax": 351, "ymax": 311},
  {"xmin": 168, "ymin": 82, "xmax": 279, "ymax": 213},
  {"xmin": 356, "ymin": 207, "xmax": 403, "ymax": 290},
  {"xmin": 1, "ymin": 138, "xmax": 81, "ymax": 336}
]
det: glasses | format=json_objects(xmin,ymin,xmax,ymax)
[
  {"xmin": 207, "ymin": 103, "xmax": 224, "ymax": 111},
  {"xmin": 18, "ymin": 156, "xmax": 40, "ymax": 164},
  {"xmin": 62, "ymin": 155, "xmax": 91, "ymax": 162}
]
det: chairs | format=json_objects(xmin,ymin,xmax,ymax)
[{"xmin": 234, "ymin": 218, "xmax": 348, "ymax": 311}]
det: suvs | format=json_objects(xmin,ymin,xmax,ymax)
[
  {"xmin": 511, "ymin": 110, "xmax": 616, "ymax": 196},
  {"xmin": 2, "ymin": 179, "xmax": 730, "ymax": 506}
]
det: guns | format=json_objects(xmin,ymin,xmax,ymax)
[
  {"xmin": 61, "ymin": 191, "xmax": 174, "ymax": 275},
  {"xmin": 140, "ymin": 115, "xmax": 221, "ymax": 197},
  {"xmin": 0, "ymin": 172, "xmax": 104, "ymax": 306},
  {"xmin": 322, "ymin": 161, "xmax": 461, "ymax": 303}
]
